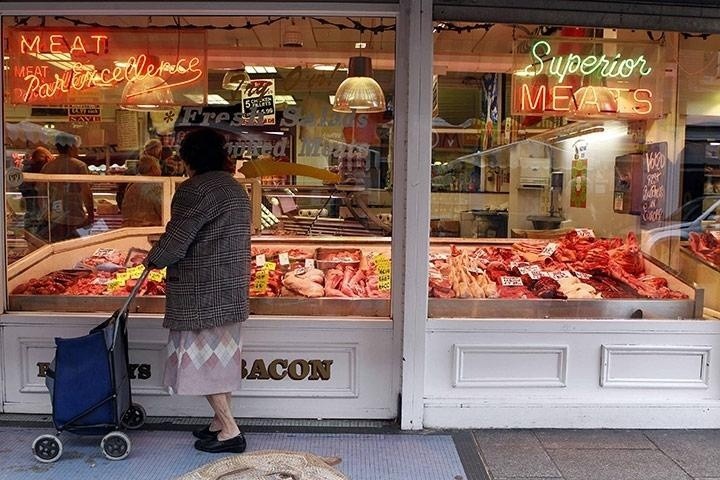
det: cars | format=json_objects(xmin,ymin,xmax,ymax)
[{"xmin": 615, "ymin": 194, "xmax": 720, "ymax": 279}]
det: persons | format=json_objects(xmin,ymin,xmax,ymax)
[
  {"xmin": 141, "ymin": 127, "xmax": 251, "ymax": 453},
  {"xmin": 19, "ymin": 133, "xmax": 188, "ymax": 244}
]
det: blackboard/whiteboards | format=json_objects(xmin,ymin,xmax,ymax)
[{"xmin": 640, "ymin": 141, "xmax": 668, "ymax": 230}]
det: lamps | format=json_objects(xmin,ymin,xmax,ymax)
[
  {"xmin": 565, "ymin": 28, "xmax": 618, "ymax": 122},
  {"xmin": 333, "ymin": 16, "xmax": 387, "ymax": 113},
  {"xmin": 282, "ymin": 17, "xmax": 304, "ymax": 48},
  {"xmin": 120, "ymin": 16, "xmax": 175, "ymax": 110}
]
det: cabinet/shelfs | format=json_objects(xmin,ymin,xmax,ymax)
[{"xmin": 1, "ymin": 158, "xmax": 720, "ymax": 429}]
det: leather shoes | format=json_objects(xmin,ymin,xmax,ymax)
[
  {"xmin": 193, "ymin": 435, "xmax": 247, "ymax": 455},
  {"xmin": 192, "ymin": 422, "xmax": 224, "ymax": 439}
]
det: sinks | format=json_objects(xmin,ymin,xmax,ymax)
[{"xmin": 525, "ymin": 215, "xmax": 565, "ymax": 230}]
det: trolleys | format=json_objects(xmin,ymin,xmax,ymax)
[{"xmin": 31, "ymin": 268, "xmax": 150, "ymax": 463}]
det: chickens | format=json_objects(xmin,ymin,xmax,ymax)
[{"xmin": 446, "ymin": 247, "xmax": 496, "ymax": 299}]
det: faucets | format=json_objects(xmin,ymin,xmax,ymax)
[{"xmin": 547, "ymin": 205, "xmax": 554, "ymax": 213}]
